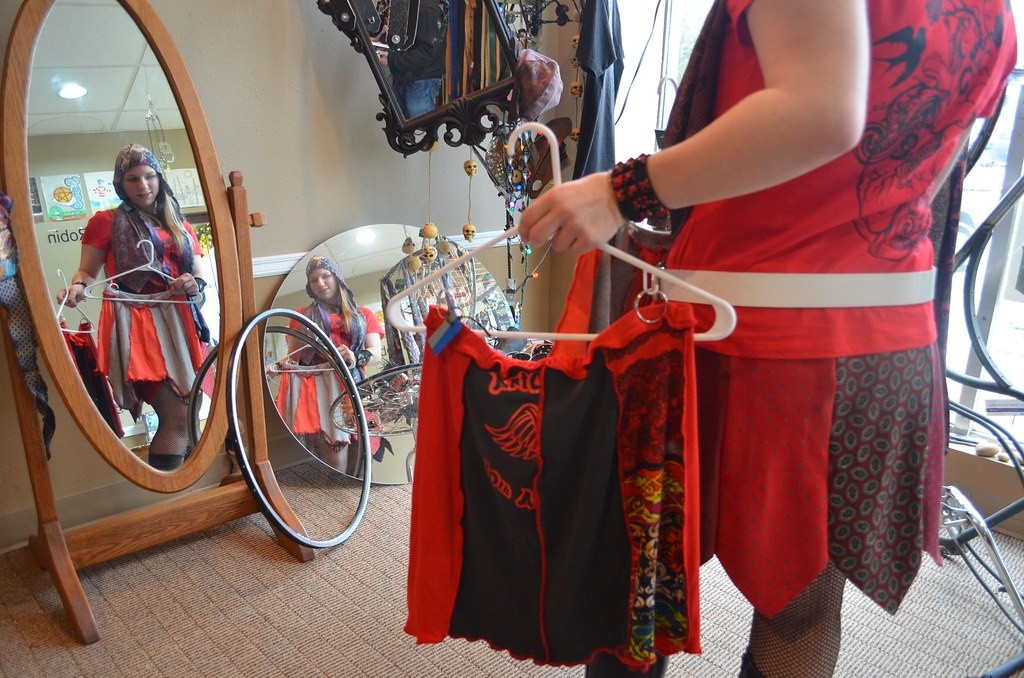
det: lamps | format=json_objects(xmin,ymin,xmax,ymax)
[{"xmin": 140, "ymin": 66, "xmax": 174, "ymax": 180}]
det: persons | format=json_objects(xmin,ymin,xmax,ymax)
[
  {"xmin": 265, "ymin": 256, "xmax": 384, "ymax": 475},
  {"xmin": 373, "ymin": 0, "xmax": 450, "ymax": 119},
  {"xmin": 517, "ymin": 0, "xmax": 1018, "ymax": 678},
  {"xmin": 56, "ymin": 144, "xmax": 207, "ymax": 471}
]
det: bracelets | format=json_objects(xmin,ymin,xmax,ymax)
[
  {"xmin": 74, "ymin": 281, "xmax": 91, "ymax": 293},
  {"xmin": 276, "ymin": 361, "xmax": 283, "ymax": 375},
  {"xmin": 611, "ymin": 154, "xmax": 670, "ymax": 223}
]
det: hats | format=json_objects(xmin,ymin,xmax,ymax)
[
  {"xmin": 305, "ymin": 255, "xmax": 352, "ymax": 298},
  {"xmin": 113, "ymin": 144, "xmax": 175, "ymax": 203}
]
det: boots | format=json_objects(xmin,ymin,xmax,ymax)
[
  {"xmin": 148, "ymin": 453, "xmax": 184, "ymax": 472},
  {"xmin": 585, "ymin": 653, "xmax": 669, "ymax": 678},
  {"xmin": 183, "ymin": 446, "xmax": 191, "ymax": 460},
  {"xmin": 739, "ymin": 642, "xmax": 764, "ymax": 678}
]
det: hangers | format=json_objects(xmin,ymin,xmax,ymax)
[
  {"xmin": 385, "ymin": 121, "xmax": 736, "ymax": 341},
  {"xmin": 53, "ymin": 270, "xmax": 96, "ymax": 333},
  {"xmin": 268, "ymin": 323, "xmax": 356, "ymax": 373},
  {"xmin": 83, "ymin": 239, "xmax": 203, "ymax": 303}
]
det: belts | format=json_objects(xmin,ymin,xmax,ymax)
[{"xmin": 661, "ymin": 266, "xmax": 937, "ymax": 308}]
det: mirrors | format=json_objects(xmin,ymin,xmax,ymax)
[
  {"xmin": 316, "ymin": 0, "xmax": 525, "ymax": 155},
  {"xmin": 262, "ymin": 224, "xmax": 517, "ymax": 487},
  {"xmin": 0, "ymin": 0, "xmax": 315, "ymax": 647}
]
